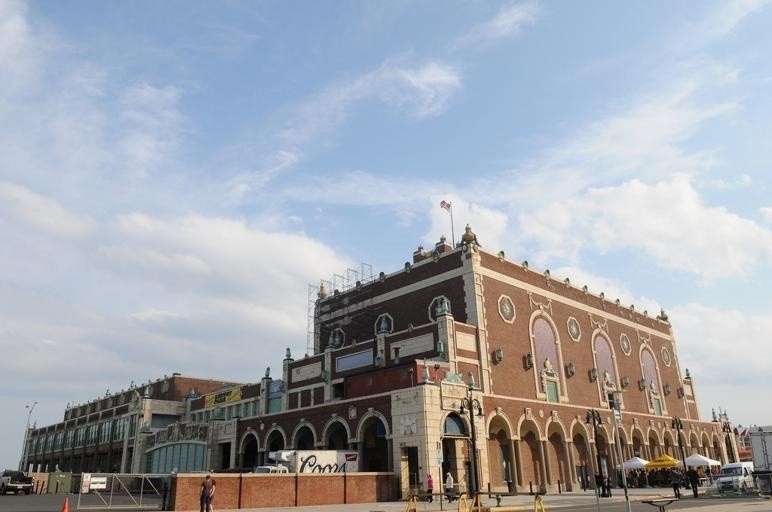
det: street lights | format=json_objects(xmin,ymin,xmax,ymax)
[
  {"xmin": 18, "ymin": 401, "xmax": 39, "ymax": 470},
  {"xmin": 671, "ymin": 414, "xmax": 692, "ymax": 489},
  {"xmin": 459, "ymin": 371, "xmax": 487, "ymax": 498},
  {"xmin": 583, "ymin": 409, "xmax": 608, "ymax": 497}
]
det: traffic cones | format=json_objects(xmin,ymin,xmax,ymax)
[{"xmin": 60, "ymin": 495, "xmax": 69, "ymax": 512}]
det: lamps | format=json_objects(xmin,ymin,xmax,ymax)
[{"xmin": 494, "ymin": 346, "xmax": 685, "ymax": 399}]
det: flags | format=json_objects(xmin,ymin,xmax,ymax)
[{"xmin": 439, "ymin": 201, "xmax": 451, "ymax": 211}]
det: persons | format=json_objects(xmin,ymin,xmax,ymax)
[
  {"xmin": 593, "ymin": 464, "xmax": 723, "ymax": 500},
  {"xmin": 443, "ymin": 471, "xmax": 454, "ymax": 503},
  {"xmin": 198, "ymin": 475, "xmax": 212, "ymax": 512},
  {"xmin": 208, "ymin": 478, "xmax": 216, "ymax": 512},
  {"xmin": 424, "ymin": 472, "xmax": 433, "ymax": 504}
]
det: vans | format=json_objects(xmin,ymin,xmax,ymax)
[{"xmin": 715, "ymin": 461, "xmax": 759, "ymax": 493}]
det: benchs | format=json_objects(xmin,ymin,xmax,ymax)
[
  {"xmin": 642, "ymin": 497, "xmax": 679, "ymax": 512},
  {"xmin": 404, "ymin": 492, "xmax": 469, "ymax": 512},
  {"xmin": 469, "ymin": 492, "xmax": 546, "ymax": 512}
]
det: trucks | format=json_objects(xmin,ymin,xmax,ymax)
[
  {"xmin": 255, "ymin": 449, "xmax": 360, "ymax": 473},
  {"xmin": 748, "ymin": 425, "xmax": 772, "ymax": 495}
]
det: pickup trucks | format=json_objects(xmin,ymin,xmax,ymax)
[{"xmin": 0, "ymin": 470, "xmax": 35, "ymax": 496}]
